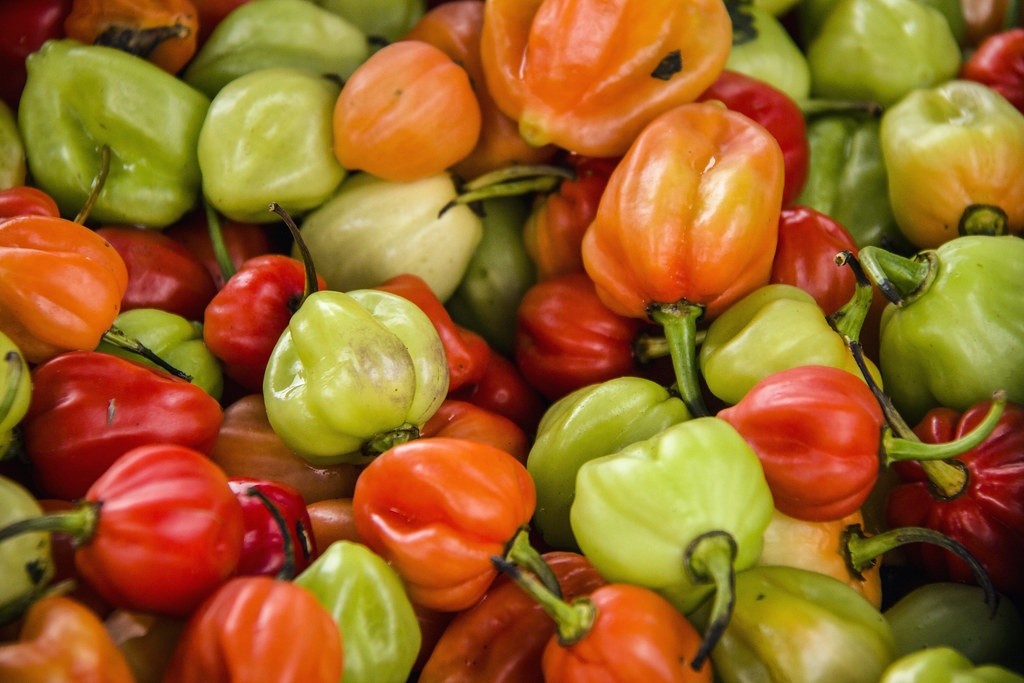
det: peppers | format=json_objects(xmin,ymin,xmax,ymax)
[{"xmin": 0, "ymin": 0, "xmax": 1024, "ymax": 683}]
